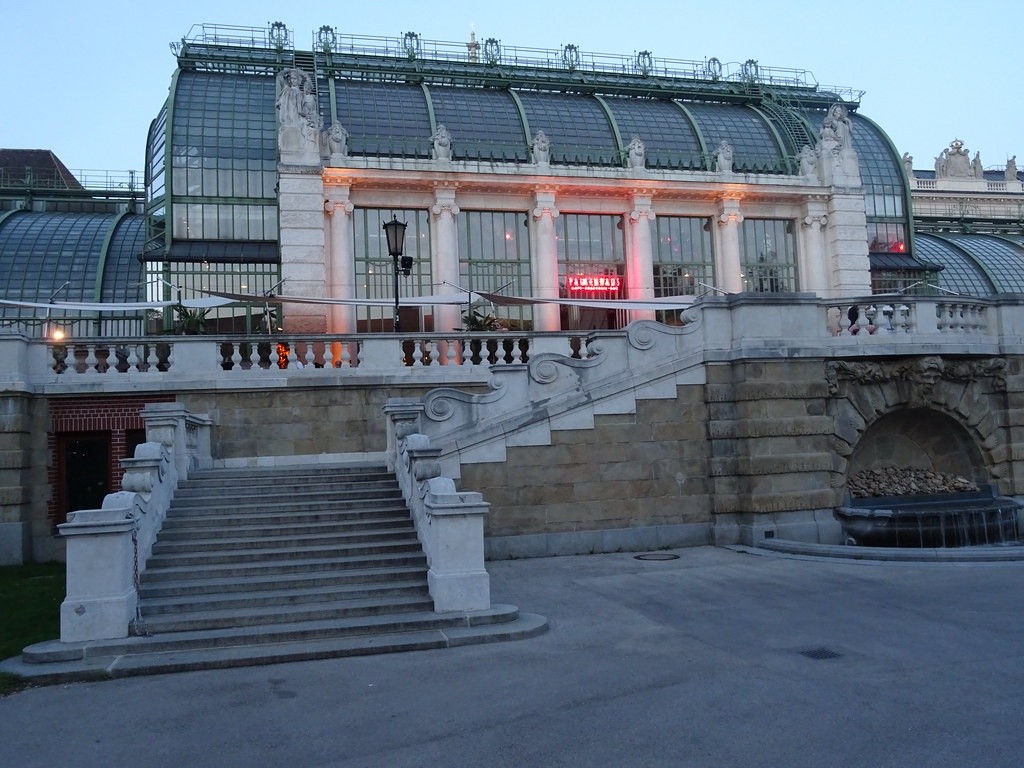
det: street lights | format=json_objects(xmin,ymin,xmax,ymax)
[{"xmin": 383, "ymin": 213, "xmax": 413, "ymax": 331}]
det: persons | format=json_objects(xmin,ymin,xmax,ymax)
[
  {"xmin": 276, "ymin": 68, "xmax": 319, "ymax": 128},
  {"xmin": 820, "ymin": 103, "xmax": 853, "ymax": 155}
]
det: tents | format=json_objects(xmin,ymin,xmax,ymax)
[
  {"xmin": 472, "ymin": 283, "xmax": 698, "ymax": 334},
  {"xmin": 1, "ymin": 280, "xmax": 259, "ymax": 334},
  {"xmin": 192, "ymin": 272, "xmax": 499, "ymax": 330}
]
